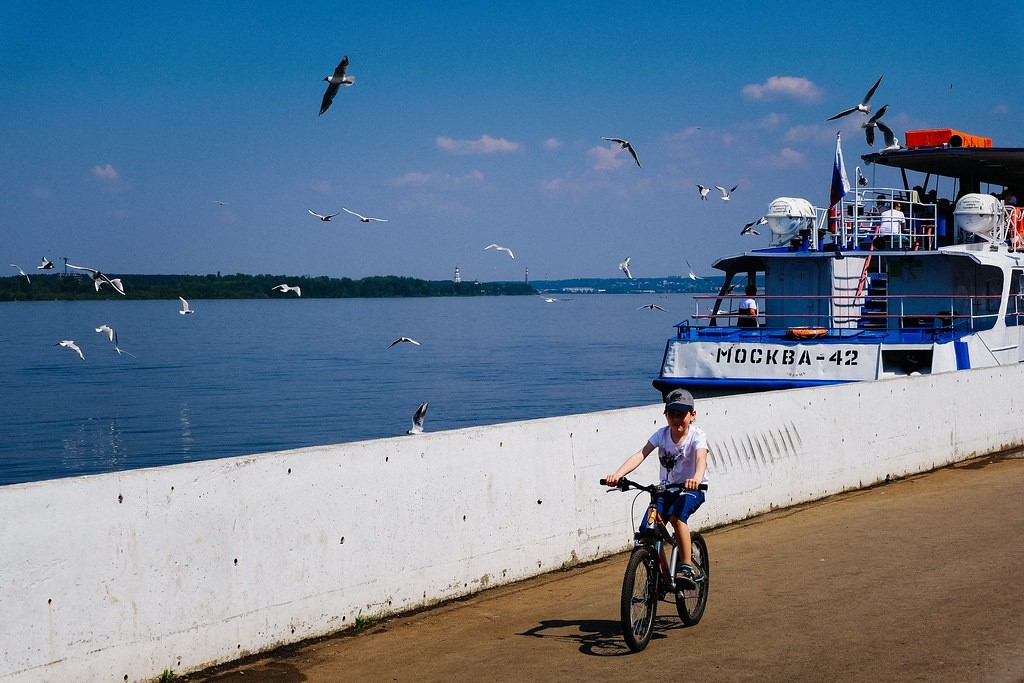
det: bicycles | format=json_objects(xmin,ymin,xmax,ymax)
[{"xmin": 599, "ymin": 475, "xmax": 709, "ymax": 655}]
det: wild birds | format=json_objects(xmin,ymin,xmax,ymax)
[
  {"xmin": 53, "ymin": 340, "xmax": 85, "ymax": 361},
  {"xmin": 483, "ymin": 244, "xmax": 515, "ymax": 260},
  {"xmin": 212, "ymin": 200, "xmax": 229, "ymax": 205},
  {"xmin": 740, "ymin": 216, "xmax": 769, "ymax": 236},
  {"xmin": 635, "ymin": 303, "xmax": 668, "ymax": 313},
  {"xmin": 705, "ymin": 304, "xmax": 739, "ymax": 315},
  {"xmin": 95, "ymin": 325, "xmax": 137, "ymax": 359},
  {"xmin": 536, "ymin": 294, "xmax": 574, "ymax": 303},
  {"xmin": 403, "ymin": 401, "xmax": 429, "ymax": 436},
  {"xmin": 36, "ymin": 256, "xmax": 56, "ymax": 269},
  {"xmin": 386, "ymin": 336, "xmax": 422, "ymax": 350},
  {"xmin": 178, "ymin": 296, "xmax": 194, "ymax": 315},
  {"xmin": 824, "ymin": 74, "xmax": 904, "ymax": 156},
  {"xmin": 318, "ymin": 55, "xmax": 356, "ymax": 116},
  {"xmin": 600, "ymin": 136, "xmax": 642, "ymax": 168},
  {"xmin": 696, "ymin": 184, "xmax": 739, "ymax": 204},
  {"xmin": 617, "ymin": 256, "xmax": 632, "ymax": 280},
  {"xmin": 65, "ymin": 262, "xmax": 125, "ymax": 295},
  {"xmin": 342, "ymin": 207, "xmax": 390, "ymax": 223},
  {"xmin": 308, "ymin": 209, "xmax": 340, "ymax": 221},
  {"xmin": 271, "ymin": 284, "xmax": 301, "ymax": 299},
  {"xmin": 9, "ymin": 263, "xmax": 31, "ymax": 285},
  {"xmin": 688, "ymin": 270, "xmax": 704, "ymax": 281}
]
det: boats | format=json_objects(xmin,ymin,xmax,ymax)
[
  {"xmin": 763, "ymin": 196, "xmax": 817, "ymax": 235},
  {"xmin": 952, "ymin": 192, "xmax": 1002, "ymax": 234},
  {"xmin": 653, "ymin": 129, "xmax": 1023, "ymax": 404}
]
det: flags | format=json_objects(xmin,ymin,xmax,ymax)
[{"xmin": 829, "ymin": 134, "xmax": 852, "ymax": 207}]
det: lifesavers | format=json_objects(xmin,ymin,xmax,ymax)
[
  {"xmin": 786, "ymin": 325, "xmax": 829, "ymax": 340},
  {"xmin": 1010, "ymin": 210, "xmax": 1024, "ymax": 248}
]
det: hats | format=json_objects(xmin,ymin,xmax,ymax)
[{"xmin": 665, "ymin": 387, "xmax": 694, "ymax": 411}]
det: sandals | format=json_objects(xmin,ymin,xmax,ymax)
[{"xmin": 674, "ymin": 564, "xmax": 697, "ymax": 590}]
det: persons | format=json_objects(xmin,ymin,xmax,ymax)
[
  {"xmin": 879, "ymin": 199, "xmax": 909, "ymax": 249},
  {"xmin": 737, "ymin": 286, "xmax": 761, "ymax": 328},
  {"xmin": 990, "ymin": 186, "xmax": 1017, "ymax": 238},
  {"xmin": 913, "ymin": 184, "xmax": 954, "ymax": 246},
  {"xmin": 867, "ymin": 193, "xmax": 888, "ymax": 224},
  {"xmin": 606, "ymin": 389, "xmax": 710, "ymax": 591}
]
